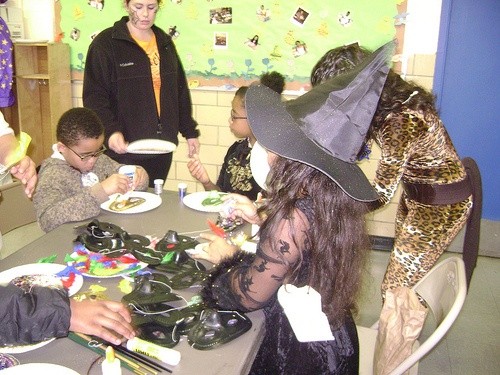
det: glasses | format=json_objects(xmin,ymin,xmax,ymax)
[
  {"xmin": 231, "ymin": 110, "xmax": 248, "ymax": 120},
  {"xmin": 65, "ymin": 145, "xmax": 108, "ymax": 162}
]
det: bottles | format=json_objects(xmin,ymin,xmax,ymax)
[
  {"xmin": 119, "ymin": 165, "xmax": 136, "ymax": 197},
  {"xmin": 177, "ymin": 182, "xmax": 188, "ymax": 198},
  {"xmin": 101, "ymin": 345, "xmax": 121, "ymax": 375},
  {"xmin": 153, "ymin": 178, "xmax": 164, "ymax": 194}
]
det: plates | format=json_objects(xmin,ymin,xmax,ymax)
[
  {"xmin": 126, "ymin": 137, "xmax": 176, "ymax": 155},
  {"xmin": 0, "ymin": 323, "xmax": 60, "ymax": 354},
  {"xmin": 67, "ymin": 239, "xmax": 153, "ymax": 278},
  {"xmin": 101, "ymin": 191, "xmax": 162, "ymax": 214},
  {"xmin": 195, "ymin": 240, "xmax": 257, "ymax": 262},
  {"xmin": 182, "ymin": 191, "xmax": 235, "ymax": 211},
  {"xmin": 0, "ymin": 263, "xmax": 84, "ymax": 299},
  {"xmin": 0, "ymin": 362, "xmax": 78, "ymax": 375}
]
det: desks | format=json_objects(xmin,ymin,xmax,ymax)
[{"xmin": 0, "ymin": 188, "xmax": 267, "ymax": 375}]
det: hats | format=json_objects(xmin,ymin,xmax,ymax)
[{"xmin": 245, "ymin": 39, "xmax": 395, "ymax": 202}]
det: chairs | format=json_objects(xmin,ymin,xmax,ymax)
[{"xmin": 356, "ymin": 257, "xmax": 466, "ymax": 375}]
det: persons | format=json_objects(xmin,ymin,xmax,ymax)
[
  {"xmin": 188, "ymin": 70, "xmax": 286, "ymax": 201},
  {"xmin": 33, "ymin": 108, "xmax": 149, "ymax": 233},
  {"xmin": 191, "ymin": 38, "xmax": 401, "ymax": 375},
  {"xmin": 0, "ymin": 282, "xmax": 137, "ymax": 346},
  {"xmin": 310, "ymin": 42, "xmax": 473, "ymax": 329},
  {"xmin": 0, "ymin": 109, "xmax": 38, "ymax": 200},
  {"xmin": 82, "ymin": 0, "xmax": 201, "ymax": 188}
]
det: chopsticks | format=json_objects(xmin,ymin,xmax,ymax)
[{"xmin": 68, "ymin": 330, "xmax": 172, "ymax": 375}]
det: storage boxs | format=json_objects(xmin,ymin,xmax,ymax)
[{"xmin": 0, "ymin": 6, "xmax": 25, "ymax": 41}]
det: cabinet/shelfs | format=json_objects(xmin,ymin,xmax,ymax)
[{"xmin": 0, "ymin": 43, "xmax": 72, "ymax": 166}]
